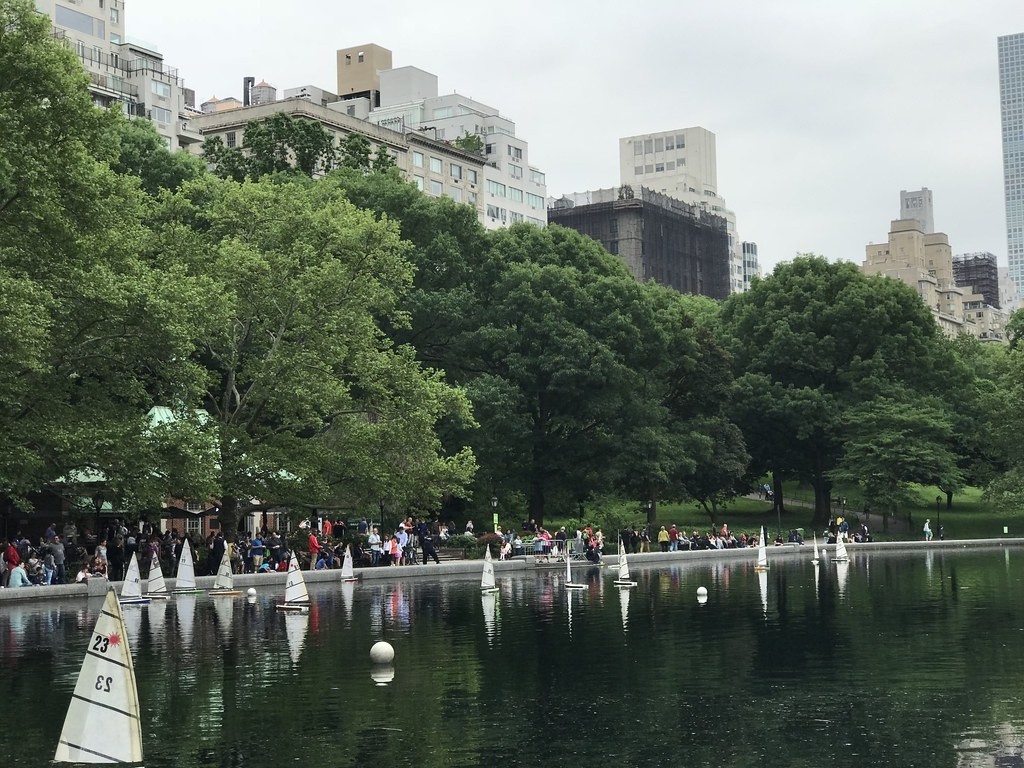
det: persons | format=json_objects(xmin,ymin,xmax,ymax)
[
  {"xmin": 835, "ymin": 495, "xmax": 848, "ymax": 507},
  {"xmin": 757, "ymin": 483, "xmax": 763, "ymax": 500},
  {"xmin": 764, "ymin": 481, "xmax": 772, "ymax": 500},
  {"xmin": 923, "ymin": 518, "xmax": 934, "ymax": 541},
  {"xmin": 0, "ymin": 516, "xmax": 476, "ymax": 588},
  {"xmin": 864, "ymin": 501, "xmax": 870, "ymax": 519},
  {"xmin": 939, "ymin": 524, "xmax": 945, "ymax": 541},
  {"xmin": 496, "ymin": 516, "xmax": 874, "ymax": 564}
]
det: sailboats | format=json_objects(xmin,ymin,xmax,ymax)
[
  {"xmin": 275, "ymin": 549, "xmax": 312, "ymax": 611},
  {"xmin": 565, "ymin": 585, "xmax": 637, "ymax": 638},
  {"xmin": 212, "ymin": 596, "xmax": 234, "ymax": 649},
  {"xmin": 119, "ymin": 551, "xmax": 152, "ymax": 604},
  {"xmin": 122, "ymin": 593, "xmax": 197, "ymax": 667},
  {"xmin": 754, "ymin": 525, "xmax": 770, "ymax": 569},
  {"xmin": 172, "ymin": 538, "xmax": 206, "ymax": 595},
  {"xmin": 755, "ymin": 569, "xmax": 768, "ymax": 617},
  {"xmin": 564, "ymin": 539, "xmax": 638, "ymax": 587},
  {"xmin": 142, "ymin": 550, "xmax": 171, "ymax": 600},
  {"xmin": 810, "ymin": 531, "xmax": 820, "ymax": 562},
  {"xmin": 480, "ymin": 544, "xmax": 500, "ymax": 593},
  {"xmin": 208, "ymin": 540, "xmax": 244, "ymax": 596},
  {"xmin": 50, "ymin": 584, "xmax": 146, "ymax": 768},
  {"xmin": 341, "ymin": 581, "xmax": 355, "ymax": 620},
  {"xmin": 481, "ymin": 592, "xmax": 500, "ymax": 645},
  {"xmin": 811, "ymin": 561, "xmax": 850, "ymax": 599},
  {"xmin": 831, "ymin": 531, "xmax": 850, "ymax": 561},
  {"xmin": 340, "ymin": 544, "xmax": 359, "ymax": 582},
  {"xmin": 285, "ymin": 611, "xmax": 309, "ymax": 663}
]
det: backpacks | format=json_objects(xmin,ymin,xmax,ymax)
[{"xmin": 582, "ymin": 529, "xmax": 590, "ymax": 540}]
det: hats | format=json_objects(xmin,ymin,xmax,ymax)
[
  {"xmin": 498, "ymin": 526, "xmax": 501, "ymax": 528},
  {"xmin": 926, "ymin": 519, "xmax": 930, "ymax": 522},
  {"xmin": 661, "ymin": 526, "xmax": 665, "ymax": 529},
  {"xmin": 672, "ymin": 524, "xmax": 676, "ymax": 527},
  {"xmin": 561, "ymin": 527, "xmax": 565, "ymax": 530}
]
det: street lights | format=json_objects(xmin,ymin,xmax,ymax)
[
  {"xmin": 647, "ymin": 499, "xmax": 653, "ymax": 539},
  {"xmin": 842, "ymin": 496, "xmax": 847, "ymax": 517},
  {"xmin": 491, "ymin": 496, "xmax": 498, "ymax": 533},
  {"xmin": 378, "ymin": 498, "xmax": 386, "ymax": 545},
  {"xmin": 92, "ymin": 487, "xmax": 105, "ymax": 546},
  {"xmin": 936, "ymin": 495, "xmax": 942, "ymax": 536}
]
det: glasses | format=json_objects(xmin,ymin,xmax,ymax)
[{"xmin": 54, "ymin": 538, "xmax": 57, "ymax": 539}]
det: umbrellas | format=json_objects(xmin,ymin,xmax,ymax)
[
  {"xmin": 198, "ymin": 506, "xmax": 255, "ymax": 517},
  {"xmin": 160, "ymin": 505, "xmax": 198, "ymax": 530}
]
